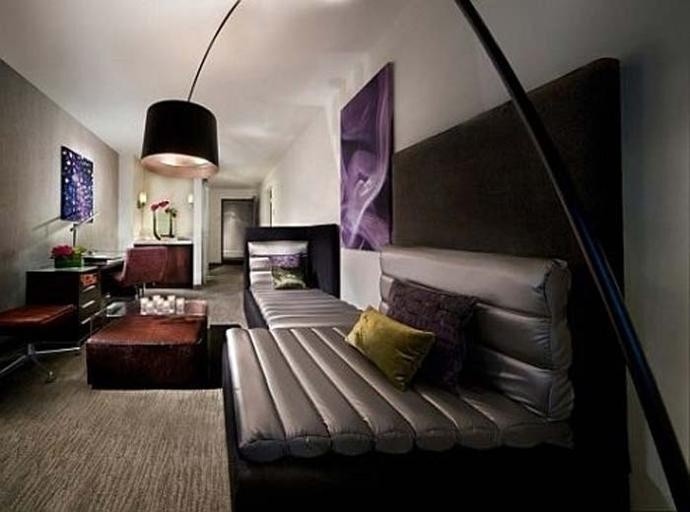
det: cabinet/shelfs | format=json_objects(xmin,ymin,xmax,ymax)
[
  {"xmin": 26, "ymin": 259, "xmax": 135, "ymax": 346},
  {"xmin": 134, "ymin": 243, "xmax": 193, "ymax": 288}
]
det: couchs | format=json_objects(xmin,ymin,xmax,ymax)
[
  {"xmin": 223, "ymin": 248, "xmax": 576, "ymax": 512},
  {"xmin": 242, "ymin": 225, "xmax": 365, "ymax": 328}
]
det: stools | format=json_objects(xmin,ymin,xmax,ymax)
[{"xmin": 1, "ymin": 304, "xmax": 81, "ymax": 383}]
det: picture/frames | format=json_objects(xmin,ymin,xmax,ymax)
[
  {"xmin": 61, "ymin": 146, "xmax": 94, "ymax": 223},
  {"xmin": 340, "ymin": 63, "xmax": 393, "ymax": 250}
]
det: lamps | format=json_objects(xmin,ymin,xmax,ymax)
[
  {"xmin": 137, "ymin": 191, "xmax": 147, "ymax": 208},
  {"xmin": 141, "ymin": 0, "xmax": 690, "ymax": 512}
]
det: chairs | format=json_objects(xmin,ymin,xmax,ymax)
[{"xmin": 106, "ymin": 247, "xmax": 167, "ymax": 301}]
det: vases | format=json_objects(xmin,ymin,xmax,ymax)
[{"xmin": 54, "ymin": 253, "xmax": 81, "ymax": 268}]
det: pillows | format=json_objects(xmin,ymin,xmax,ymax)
[
  {"xmin": 269, "ymin": 253, "xmax": 302, "ymax": 268},
  {"xmin": 386, "ymin": 278, "xmax": 478, "ymax": 397},
  {"xmin": 344, "ymin": 305, "xmax": 436, "ymax": 392},
  {"xmin": 272, "ymin": 266, "xmax": 307, "ymax": 289}
]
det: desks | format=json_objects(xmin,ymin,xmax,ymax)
[{"xmin": 83, "ymin": 256, "xmax": 123, "ymax": 283}]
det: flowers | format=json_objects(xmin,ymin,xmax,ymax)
[{"xmin": 49, "ymin": 246, "xmax": 88, "ymax": 260}]
